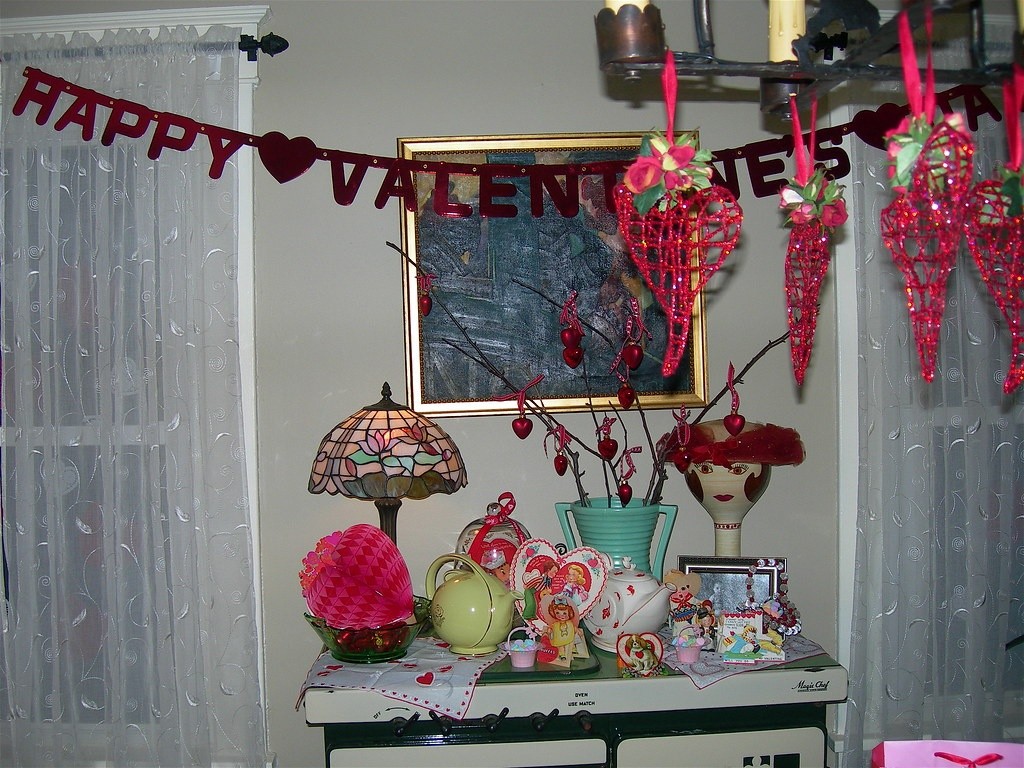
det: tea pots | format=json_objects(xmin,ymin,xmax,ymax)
[
  {"xmin": 425, "ymin": 554, "xmax": 525, "ymax": 654},
  {"xmin": 583, "ymin": 568, "xmax": 676, "ymax": 653}
]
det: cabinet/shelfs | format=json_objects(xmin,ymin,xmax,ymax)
[{"xmin": 295, "ymin": 620, "xmax": 850, "ymax": 768}]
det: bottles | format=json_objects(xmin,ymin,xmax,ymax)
[{"xmin": 454, "ymin": 503, "xmax": 532, "ymax": 591}]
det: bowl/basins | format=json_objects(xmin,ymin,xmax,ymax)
[{"xmin": 303, "ymin": 595, "xmax": 432, "ymax": 664}]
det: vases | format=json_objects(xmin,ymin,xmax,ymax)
[{"xmin": 553, "ymin": 495, "xmax": 678, "ymax": 582}]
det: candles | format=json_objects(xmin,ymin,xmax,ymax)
[
  {"xmin": 768, "ymin": 0, "xmax": 807, "ymax": 61},
  {"xmin": 604, "ymin": 0, "xmax": 650, "ymax": 14}
]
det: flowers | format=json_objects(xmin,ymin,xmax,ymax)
[
  {"xmin": 980, "ymin": 158, "xmax": 1024, "ymax": 225},
  {"xmin": 778, "ymin": 162, "xmax": 850, "ymax": 237},
  {"xmin": 623, "ymin": 126, "xmax": 719, "ymax": 216},
  {"xmin": 881, "ymin": 112, "xmax": 975, "ymax": 194}
]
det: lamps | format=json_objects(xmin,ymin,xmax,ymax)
[{"xmin": 306, "ymin": 379, "xmax": 472, "ymax": 548}]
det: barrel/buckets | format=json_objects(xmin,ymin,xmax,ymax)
[
  {"xmin": 677, "ymin": 626, "xmax": 705, "ymax": 662},
  {"xmin": 507, "ymin": 627, "xmax": 537, "ymax": 668}
]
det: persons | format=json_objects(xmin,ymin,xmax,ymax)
[{"xmin": 698, "ymin": 607, "xmax": 716, "ymax": 651}]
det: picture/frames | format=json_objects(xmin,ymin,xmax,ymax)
[
  {"xmin": 677, "ymin": 554, "xmax": 788, "ymax": 631},
  {"xmin": 396, "ymin": 129, "xmax": 711, "ymax": 417}
]
